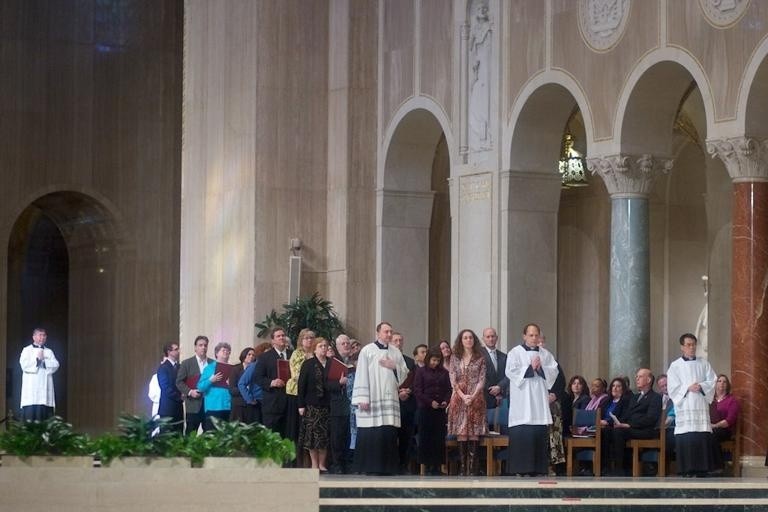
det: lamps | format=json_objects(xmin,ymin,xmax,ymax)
[{"xmin": 558, "ymin": 124, "xmax": 588, "ymax": 190}]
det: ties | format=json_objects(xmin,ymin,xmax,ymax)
[
  {"xmin": 280, "ymin": 353, "xmax": 284, "ymax": 359},
  {"xmin": 491, "ymin": 350, "xmax": 496, "ymax": 367},
  {"xmin": 174, "ymin": 362, "xmax": 177, "ymax": 368}
]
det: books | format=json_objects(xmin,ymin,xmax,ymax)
[
  {"xmin": 329, "ymin": 358, "xmax": 354, "ymax": 381},
  {"xmin": 180, "ymin": 373, "xmax": 200, "ymax": 397},
  {"xmin": 277, "ymin": 359, "xmax": 291, "ymax": 381},
  {"xmin": 214, "ymin": 362, "xmax": 244, "ymax": 388}
]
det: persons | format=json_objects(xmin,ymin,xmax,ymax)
[
  {"xmin": 566, "ymin": 378, "xmax": 610, "ymax": 475},
  {"xmin": 449, "ymin": 329, "xmax": 487, "ymax": 476},
  {"xmin": 481, "ymin": 328, "xmax": 510, "ymax": 432},
  {"xmin": 438, "ymin": 341, "xmax": 453, "ymax": 371},
  {"xmin": 148, "ymin": 353, "xmax": 168, "ymax": 438},
  {"xmin": 19, "ymin": 328, "xmax": 60, "ymax": 424},
  {"xmin": 326, "ymin": 345, "xmax": 336, "ymax": 359},
  {"xmin": 351, "ymin": 322, "xmax": 410, "ymax": 475},
  {"xmin": 227, "ymin": 347, "xmax": 256, "ymax": 421},
  {"xmin": 667, "ymin": 333, "xmax": 718, "ymax": 478},
  {"xmin": 578, "ymin": 378, "xmax": 630, "ymax": 476},
  {"xmin": 297, "ymin": 337, "xmax": 333, "ymax": 475},
  {"xmin": 390, "ymin": 333, "xmax": 415, "ymax": 475},
  {"xmin": 710, "ymin": 374, "xmax": 740, "ymax": 442},
  {"xmin": 538, "ymin": 331, "xmax": 566, "ymax": 475},
  {"xmin": 414, "ymin": 346, "xmax": 453, "ymax": 475},
  {"xmin": 505, "ymin": 324, "xmax": 559, "ymax": 477},
  {"xmin": 176, "ymin": 336, "xmax": 215, "ymax": 436},
  {"xmin": 285, "ymin": 336, "xmax": 292, "ymax": 350},
  {"xmin": 350, "ymin": 339, "xmax": 361, "ymax": 356},
  {"xmin": 254, "ymin": 327, "xmax": 293, "ymax": 435},
  {"xmin": 157, "ymin": 342, "xmax": 185, "ymax": 436},
  {"xmin": 325, "ymin": 334, "xmax": 360, "ymax": 474},
  {"xmin": 286, "ymin": 328, "xmax": 315, "ymax": 468},
  {"xmin": 413, "ymin": 344, "xmax": 427, "ymax": 370},
  {"xmin": 238, "ymin": 343, "xmax": 272, "ymax": 424},
  {"xmin": 606, "ymin": 368, "xmax": 662, "ymax": 476},
  {"xmin": 642, "ymin": 374, "xmax": 676, "ymax": 476},
  {"xmin": 569, "ymin": 375, "xmax": 592, "ymax": 410},
  {"xmin": 197, "ymin": 343, "xmax": 232, "ymax": 430}
]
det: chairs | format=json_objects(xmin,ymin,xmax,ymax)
[
  {"xmin": 549, "ymin": 408, "xmax": 603, "ymax": 477},
  {"xmin": 445, "ymin": 399, "xmax": 509, "ymax": 475},
  {"xmin": 625, "ymin": 409, "xmax": 667, "ymax": 477},
  {"xmin": 718, "ymin": 412, "xmax": 744, "ymax": 478}
]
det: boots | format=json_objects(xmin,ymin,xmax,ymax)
[
  {"xmin": 469, "ymin": 440, "xmax": 480, "ymax": 476},
  {"xmin": 456, "ymin": 441, "xmax": 467, "ymax": 476}
]
